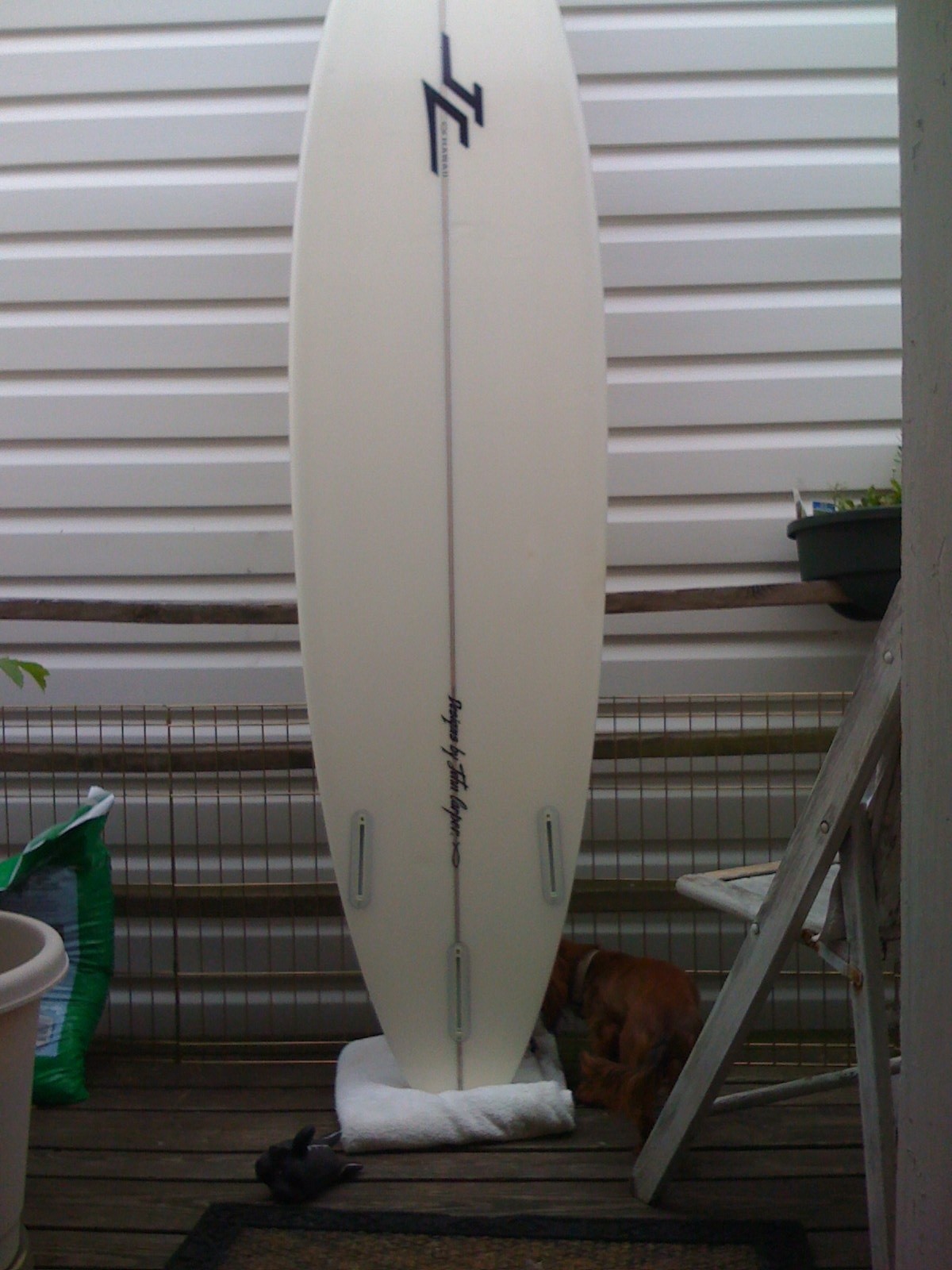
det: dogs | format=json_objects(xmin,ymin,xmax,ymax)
[{"xmin": 540, "ymin": 937, "xmax": 702, "ymax": 1145}]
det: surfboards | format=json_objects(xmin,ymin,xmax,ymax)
[{"xmin": 279, "ymin": 1, "xmax": 615, "ymax": 1095}]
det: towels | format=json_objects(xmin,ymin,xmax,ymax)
[{"xmin": 334, "ymin": 1017, "xmax": 576, "ymax": 1155}]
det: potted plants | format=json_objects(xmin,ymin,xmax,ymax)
[
  {"xmin": 0, "ymin": 656, "xmax": 70, "ymax": 1270},
  {"xmin": 785, "ymin": 435, "xmax": 903, "ymax": 581}
]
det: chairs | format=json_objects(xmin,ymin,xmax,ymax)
[{"xmin": 633, "ymin": 575, "xmax": 907, "ymax": 1270}]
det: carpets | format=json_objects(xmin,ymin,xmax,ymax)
[{"xmin": 158, "ymin": 1198, "xmax": 812, "ymax": 1270}]
